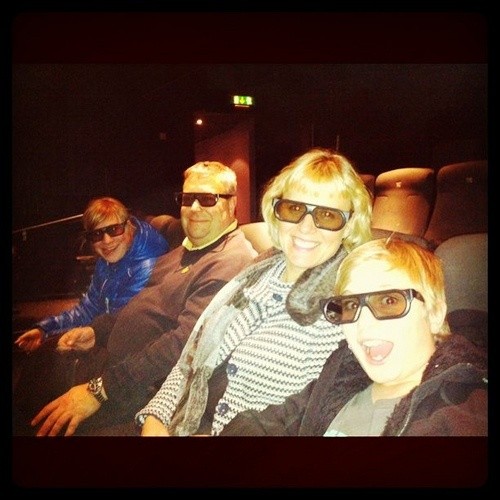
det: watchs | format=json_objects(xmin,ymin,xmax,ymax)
[{"xmin": 86, "ymin": 379, "xmax": 109, "ymax": 406}]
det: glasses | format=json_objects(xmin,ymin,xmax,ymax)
[
  {"xmin": 319, "ymin": 288, "xmax": 425, "ymax": 326},
  {"xmin": 272, "ymin": 199, "xmax": 354, "ymax": 231},
  {"xmin": 86, "ymin": 216, "xmax": 130, "ymax": 243},
  {"xmin": 173, "ymin": 191, "xmax": 234, "ymax": 207}
]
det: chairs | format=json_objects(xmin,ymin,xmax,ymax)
[{"xmin": 138, "ymin": 159, "xmax": 487, "ymax": 315}]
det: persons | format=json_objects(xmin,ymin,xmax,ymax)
[
  {"xmin": 219, "ymin": 235, "xmax": 489, "ymax": 436},
  {"xmin": 0, "ymin": 159, "xmax": 258, "ymax": 435},
  {"xmin": 133, "ymin": 151, "xmax": 374, "ymax": 436}
]
